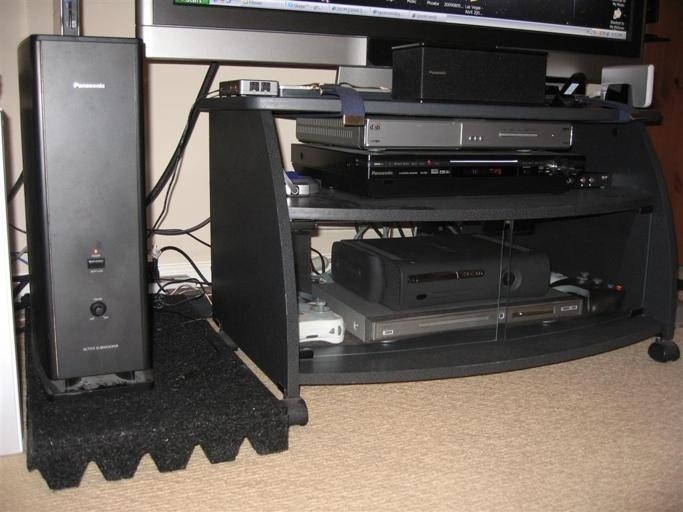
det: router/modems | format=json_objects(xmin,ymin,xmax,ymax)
[{"xmin": 218, "ymin": 79, "xmax": 279, "ymax": 99}]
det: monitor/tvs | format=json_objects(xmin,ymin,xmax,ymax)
[{"xmin": 151, "ymin": 0, "xmax": 647, "ymax": 68}]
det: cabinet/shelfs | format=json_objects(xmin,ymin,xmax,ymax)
[{"xmin": 203, "ymin": 94, "xmax": 679, "ymax": 424}]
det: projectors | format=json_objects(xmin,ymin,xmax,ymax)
[{"xmin": 332, "ymin": 231, "xmax": 552, "ymax": 312}]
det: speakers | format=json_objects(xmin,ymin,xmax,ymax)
[{"xmin": 17, "ymin": 31, "xmax": 156, "ymax": 404}]
